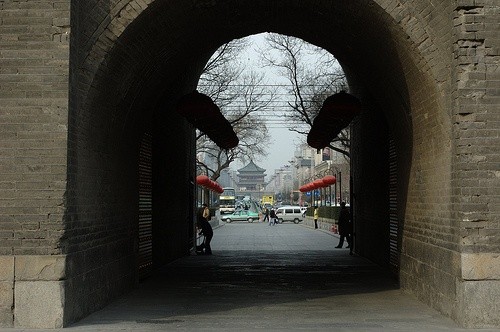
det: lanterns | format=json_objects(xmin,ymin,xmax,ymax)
[
  {"xmin": 196, "ymin": 175, "xmax": 224, "ymax": 194},
  {"xmin": 298, "ymin": 175, "xmax": 336, "ymax": 193}
]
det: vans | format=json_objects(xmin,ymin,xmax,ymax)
[{"xmin": 271, "ymin": 206, "xmax": 304, "ymax": 224}]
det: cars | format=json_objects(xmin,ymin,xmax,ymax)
[
  {"xmin": 257, "ymin": 195, "xmax": 277, "ymax": 214},
  {"xmin": 221, "ymin": 209, "xmax": 259, "ymax": 223}
]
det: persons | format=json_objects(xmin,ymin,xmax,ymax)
[
  {"xmin": 314, "ymin": 206, "xmax": 319, "ymax": 229},
  {"xmin": 197, "ymin": 217, "xmax": 213, "ymax": 255},
  {"xmin": 335, "ymin": 200, "xmax": 352, "ymax": 248},
  {"xmin": 203, "ymin": 203, "xmax": 209, "ymax": 221},
  {"xmin": 264, "ymin": 207, "xmax": 279, "ymax": 225},
  {"xmin": 235, "ymin": 203, "xmax": 248, "ymax": 210}
]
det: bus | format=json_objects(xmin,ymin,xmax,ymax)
[{"xmin": 218, "ymin": 188, "xmax": 236, "ymax": 214}]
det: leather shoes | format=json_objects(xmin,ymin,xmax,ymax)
[
  {"xmin": 335, "ymin": 246, "xmax": 342, "ymax": 248},
  {"xmin": 345, "ymin": 245, "xmax": 350, "ymax": 248}
]
